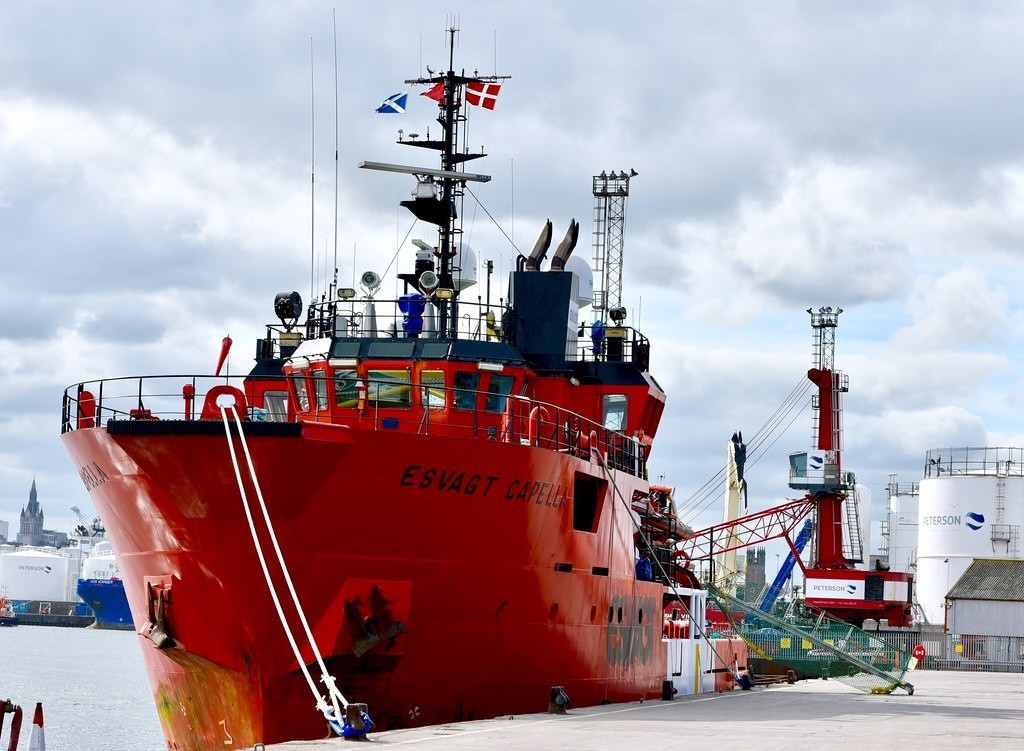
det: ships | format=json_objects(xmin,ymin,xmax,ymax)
[{"xmin": 59, "ymin": 10, "xmax": 754, "ymax": 748}]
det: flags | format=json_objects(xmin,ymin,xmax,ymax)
[
  {"xmin": 465, "ymin": 82, "xmax": 502, "ymax": 111},
  {"xmin": 419, "ymin": 80, "xmax": 446, "ymax": 105},
  {"xmin": 374, "ymin": 91, "xmax": 407, "ymax": 115}
]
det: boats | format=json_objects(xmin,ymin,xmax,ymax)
[
  {"xmin": 0, "ymin": 585, "xmax": 20, "ymax": 628},
  {"xmin": 75, "ymin": 537, "xmax": 136, "ymax": 632}
]
